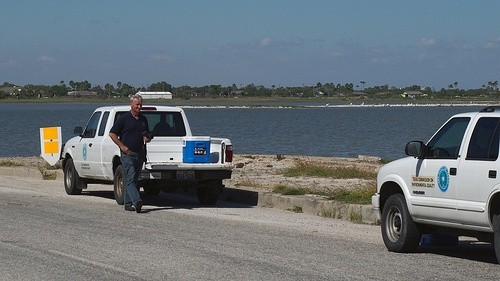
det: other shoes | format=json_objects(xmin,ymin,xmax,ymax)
[
  {"xmin": 125, "ymin": 206, "xmax": 136, "ymax": 211},
  {"xmin": 135, "ymin": 200, "xmax": 142, "ymax": 213}
]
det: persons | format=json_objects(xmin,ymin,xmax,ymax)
[{"xmin": 109, "ymin": 94, "xmax": 154, "ymax": 213}]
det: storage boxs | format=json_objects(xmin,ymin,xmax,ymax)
[{"xmin": 182, "ymin": 136, "xmax": 211, "ymax": 163}]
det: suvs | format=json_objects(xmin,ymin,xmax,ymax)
[{"xmin": 371, "ymin": 104, "xmax": 500, "ymax": 262}]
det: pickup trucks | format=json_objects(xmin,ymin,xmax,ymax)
[{"xmin": 60, "ymin": 106, "xmax": 234, "ymax": 207}]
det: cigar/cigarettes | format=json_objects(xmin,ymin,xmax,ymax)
[{"xmin": 144, "ymin": 140, "xmax": 146, "ymax": 144}]
